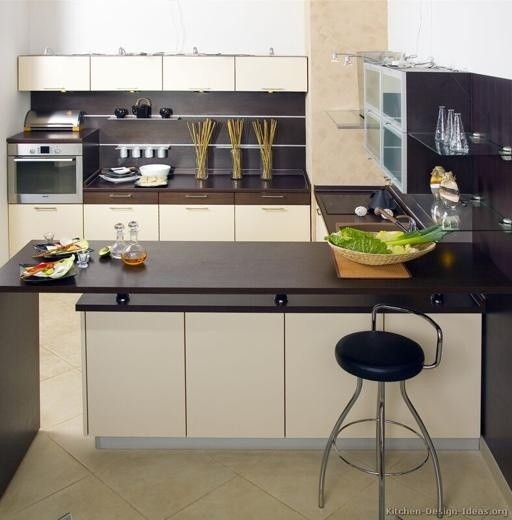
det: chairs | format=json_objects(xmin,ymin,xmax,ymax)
[{"xmin": 317, "ymin": 302, "xmax": 444, "ymax": 519}]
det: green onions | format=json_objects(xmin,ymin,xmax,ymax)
[{"xmin": 384, "ymin": 224, "xmax": 447, "ymax": 246}]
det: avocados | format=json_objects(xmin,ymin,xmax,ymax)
[{"xmin": 99, "ymin": 245, "xmax": 112, "ymax": 257}]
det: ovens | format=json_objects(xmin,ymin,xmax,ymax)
[{"xmin": 6, "ymin": 156, "xmax": 84, "ymax": 204}]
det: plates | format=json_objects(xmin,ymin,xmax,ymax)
[
  {"xmin": 18, "ymin": 258, "xmax": 80, "ymax": 283},
  {"xmin": 36, "ymin": 240, "xmax": 92, "ymax": 260},
  {"xmin": 134, "ymin": 179, "xmax": 168, "ymax": 187},
  {"xmin": 98, "ymin": 167, "xmax": 140, "ymax": 183}
]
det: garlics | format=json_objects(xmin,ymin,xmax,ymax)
[
  {"xmin": 381, "ymin": 209, "xmax": 393, "ymax": 219},
  {"xmin": 355, "ymin": 206, "xmax": 367, "ymax": 217}
]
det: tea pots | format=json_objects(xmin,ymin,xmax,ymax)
[{"xmin": 132, "ymin": 98, "xmax": 152, "ymax": 118}]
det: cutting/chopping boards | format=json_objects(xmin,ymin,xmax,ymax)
[{"xmin": 330, "ymin": 249, "xmax": 413, "ymax": 280}]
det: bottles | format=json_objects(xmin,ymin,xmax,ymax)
[
  {"xmin": 430, "ymin": 164, "xmax": 460, "ymax": 209},
  {"xmin": 122, "ymin": 221, "xmax": 147, "ymax": 266},
  {"xmin": 433, "ymin": 103, "xmax": 472, "ymax": 154},
  {"xmin": 111, "ymin": 222, "xmax": 128, "ymax": 259}
]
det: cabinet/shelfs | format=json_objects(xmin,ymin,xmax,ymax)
[
  {"xmin": 158, "ymin": 191, "xmax": 234, "ymax": 242},
  {"xmin": 84, "ymin": 191, "xmax": 158, "ymax": 243},
  {"xmin": 72, "ymin": 291, "xmax": 484, "ymax": 452},
  {"xmin": 361, "ymin": 61, "xmax": 469, "ymax": 196},
  {"xmin": 405, "ymin": 131, "xmax": 512, "ymax": 232},
  {"xmin": 18, "ymin": 54, "xmax": 308, "ymax": 93},
  {"xmin": 234, "ymin": 191, "xmax": 310, "ymax": 244},
  {"xmin": 8, "ymin": 204, "xmax": 84, "ymax": 259},
  {"xmin": 312, "ymin": 189, "xmax": 334, "ymax": 243}
]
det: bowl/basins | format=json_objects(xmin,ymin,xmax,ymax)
[{"xmin": 140, "ymin": 164, "xmax": 171, "ymax": 177}]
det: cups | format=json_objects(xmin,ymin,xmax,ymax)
[
  {"xmin": 114, "ymin": 108, "xmax": 126, "ymax": 118},
  {"xmin": 44, "ymin": 232, "xmax": 56, "ymax": 243},
  {"xmin": 160, "ymin": 109, "xmax": 172, "ymax": 118},
  {"xmin": 77, "ymin": 250, "xmax": 90, "ymax": 269}
]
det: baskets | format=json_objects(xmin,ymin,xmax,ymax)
[{"xmin": 326, "ymin": 230, "xmax": 436, "ymax": 266}]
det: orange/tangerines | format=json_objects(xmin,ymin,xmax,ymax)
[{"xmin": 438, "ymin": 250, "xmax": 457, "ymax": 270}]
